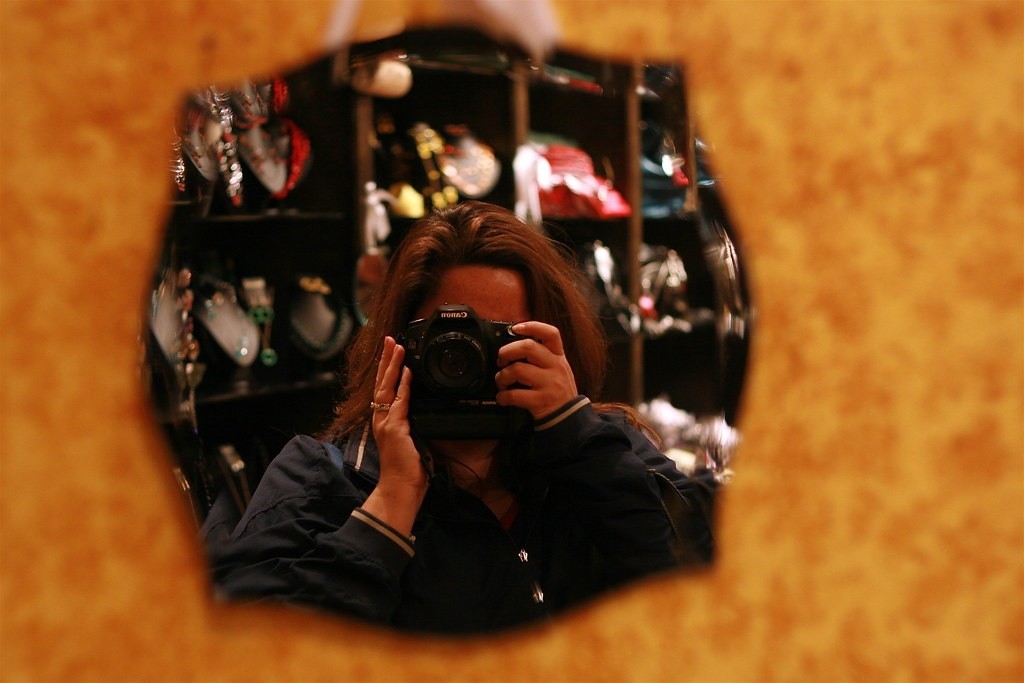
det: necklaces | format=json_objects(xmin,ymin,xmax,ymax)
[
  {"xmin": 233, "ymin": 85, "xmax": 265, "ymax": 122},
  {"xmin": 206, "ymin": 302, "xmax": 251, "ymax": 360},
  {"xmin": 185, "ymin": 138, "xmax": 215, "ymax": 174},
  {"xmin": 293, "ymin": 307, "xmax": 348, "ymax": 351},
  {"xmin": 249, "ymin": 304, "xmax": 277, "ymax": 366},
  {"xmin": 243, "ymin": 135, "xmax": 280, "ymax": 181}
]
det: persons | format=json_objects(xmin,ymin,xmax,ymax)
[{"xmin": 226, "ymin": 203, "xmax": 717, "ymax": 639}]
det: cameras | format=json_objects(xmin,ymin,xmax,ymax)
[{"xmin": 396, "ymin": 302, "xmax": 538, "ymax": 410}]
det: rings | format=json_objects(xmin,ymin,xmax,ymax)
[{"xmin": 369, "ymin": 401, "xmax": 390, "ymax": 411}]
